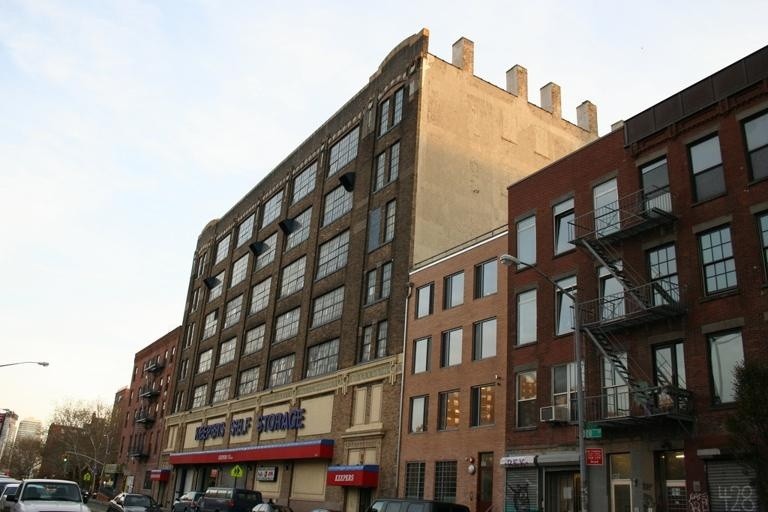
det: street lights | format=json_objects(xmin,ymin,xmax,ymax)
[
  {"xmin": 0, "ymin": 361, "xmax": 49, "ymax": 367},
  {"xmin": 63, "ymin": 450, "xmax": 103, "ymax": 466},
  {"xmin": 499, "ymin": 254, "xmax": 589, "ymax": 511}
]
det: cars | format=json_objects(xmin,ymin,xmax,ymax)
[
  {"xmin": 106, "ymin": 492, "xmax": 163, "ymax": 512},
  {"xmin": 171, "ymin": 487, "xmax": 294, "ymax": 512},
  {"xmin": 364, "ymin": 499, "xmax": 470, "ymax": 512},
  {"xmin": 0, "ymin": 474, "xmax": 93, "ymax": 512}
]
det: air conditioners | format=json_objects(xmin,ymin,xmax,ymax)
[{"xmin": 540, "ymin": 406, "xmax": 567, "ymax": 423}]
